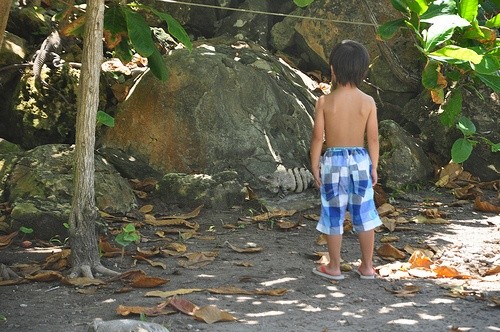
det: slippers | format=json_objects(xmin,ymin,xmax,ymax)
[
  {"xmin": 313, "ymin": 265, "xmax": 344, "ymax": 280},
  {"xmin": 356, "ymin": 264, "xmax": 375, "ymax": 279}
]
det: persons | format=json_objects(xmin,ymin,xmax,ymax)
[{"xmin": 308, "ymin": 39, "xmax": 383, "ymax": 280}]
